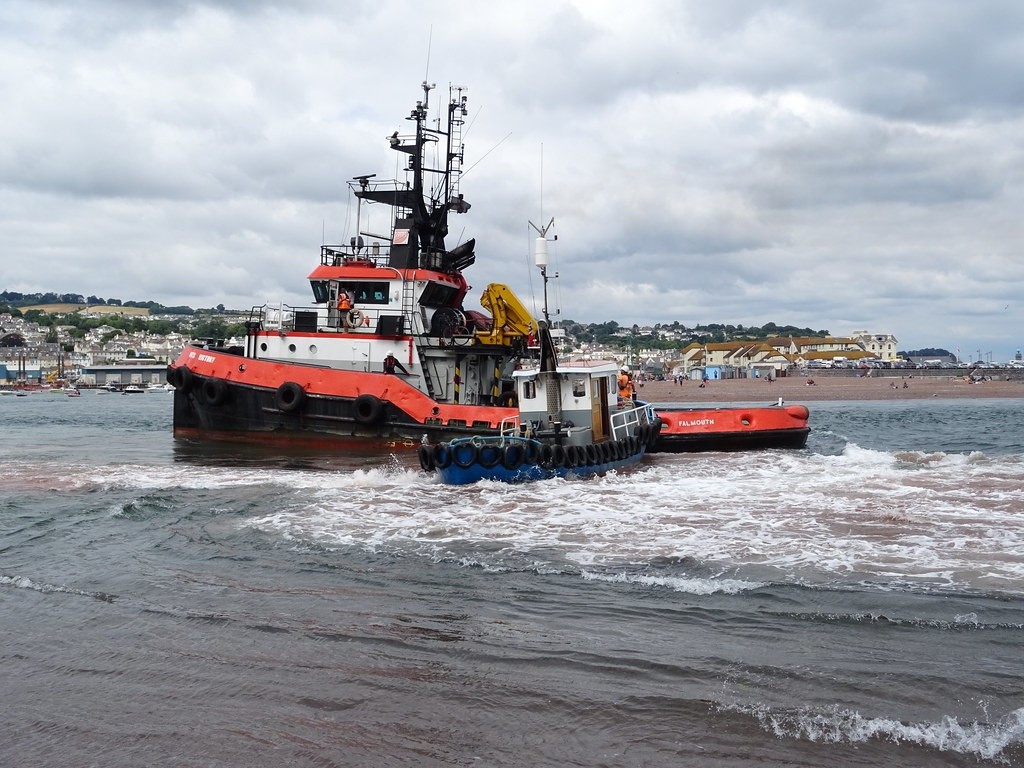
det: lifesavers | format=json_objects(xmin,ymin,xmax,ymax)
[{"xmin": 345, "ymin": 310, "xmax": 363, "ymax": 327}]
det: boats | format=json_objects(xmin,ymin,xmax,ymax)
[
  {"xmin": 0, "ymin": 381, "xmax": 177, "ymax": 400},
  {"xmin": 157, "ymin": 19, "xmax": 814, "ymax": 458},
  {"xmin": 420, "ymin": 141, "xmax": 668, "ymax": 491}
]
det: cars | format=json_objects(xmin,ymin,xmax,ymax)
[{"xmin": 800, "ymin": 348, "xmax": 1024, "ymax": 379}]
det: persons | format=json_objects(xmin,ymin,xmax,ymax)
[
  {"xmin": 617, "ymin": 365, "xmax": 633, "ymax": 408},
  {"xmin": 384, "ymin": 351, "xmax": 411, "ymax": 376},
  {"xmin": 338, "ymin": 287, "xmax": 351, "ymax": 335},
  {"xmin": 346, "ymin": 287, "xmax": 355, "ymax": 327},
  {"xmin": 627, "ymin": 371, "xmax": 637, "ymax": 400},
  {"xmin": 639, "ymin": 360, "xmax": 1003, "ymax": 404},
  {"xmin": 67, "ymin": 387, "xmax": 130, "ymax": 398}
]
